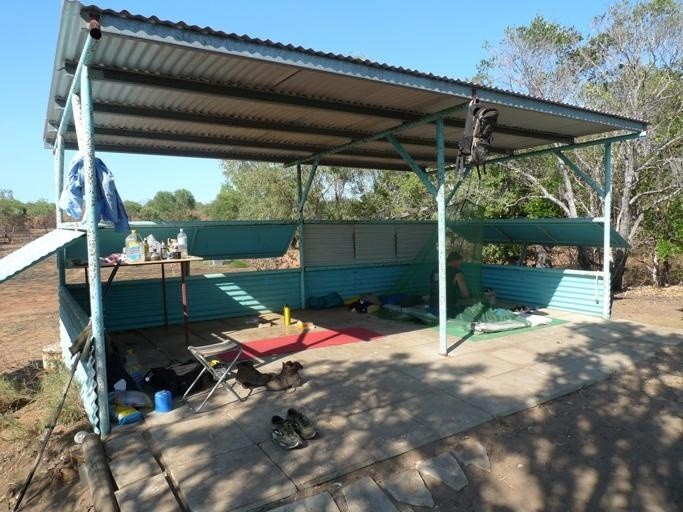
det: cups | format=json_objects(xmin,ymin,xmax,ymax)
[{"xmin": 172, "ymin": 252, "xmax": 181, "ymax": 259}]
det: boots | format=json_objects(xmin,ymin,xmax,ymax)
[
  {"xmin": 236, "ymin": 361, "xmax": 270, "ymax": 388},
  {"xmin": 265, "ymin": 362, "xmax": 302, "ymax": 391}
]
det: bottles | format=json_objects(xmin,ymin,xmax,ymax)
[
  {"xmin": 143, "ymin": 238, "xmax": 149, "ymax": 253},
  {"xmin": 160, "ymin": 240, "xmax": 167, "ymax": 259},
  {"xmin": 176, "ymin": 228, "xmax": 187, "ymax": 257},
  {"xmin": 283, "ymin": 304, "xmax": 291, "ymax": 326}
]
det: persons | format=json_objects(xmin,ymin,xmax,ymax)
[{"xmin": 427, "ymin": 251, "xmax": 472, "ymax": 319}]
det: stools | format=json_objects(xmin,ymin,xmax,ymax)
[{"xmin": 181, "ymin": 339, "xmax": 252, "ymax": 412}]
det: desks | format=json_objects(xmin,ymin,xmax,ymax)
[{"xmin": 73, "ymin": 255, "xmax": 203, "ymax": 352}]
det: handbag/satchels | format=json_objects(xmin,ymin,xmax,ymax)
[{"xmin": 146, "ymin": 359, "xmax": 211, "ymax": 396}]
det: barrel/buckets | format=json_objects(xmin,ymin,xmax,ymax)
[
  {"xmin": 124, "ymin": 228, "xmax": 143, "ymax": 251},
  {"xmin": 484, "ymin": 291, "xmax": 493, "ymax": 304}
]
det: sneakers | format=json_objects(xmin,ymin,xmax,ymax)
[
  {"xmin": 287, "ymin": 408, "xmax": 317, "ymax": 439},
  {"xmin": 271, "ymin": 415, "xmax": 302, "ymax": 450}
]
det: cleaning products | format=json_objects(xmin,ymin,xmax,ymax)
[{"xmin": 123, "ymin": 342, "xmax": 144, "ymax": 391}]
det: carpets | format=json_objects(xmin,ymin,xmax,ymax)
[
  {"xmin": 374, "ymin": 306, "xmax": 571, "ymax": 343},
  {"xmin": 214, "ymin": 327, "xmax": 383, "ymax": 364}
]
